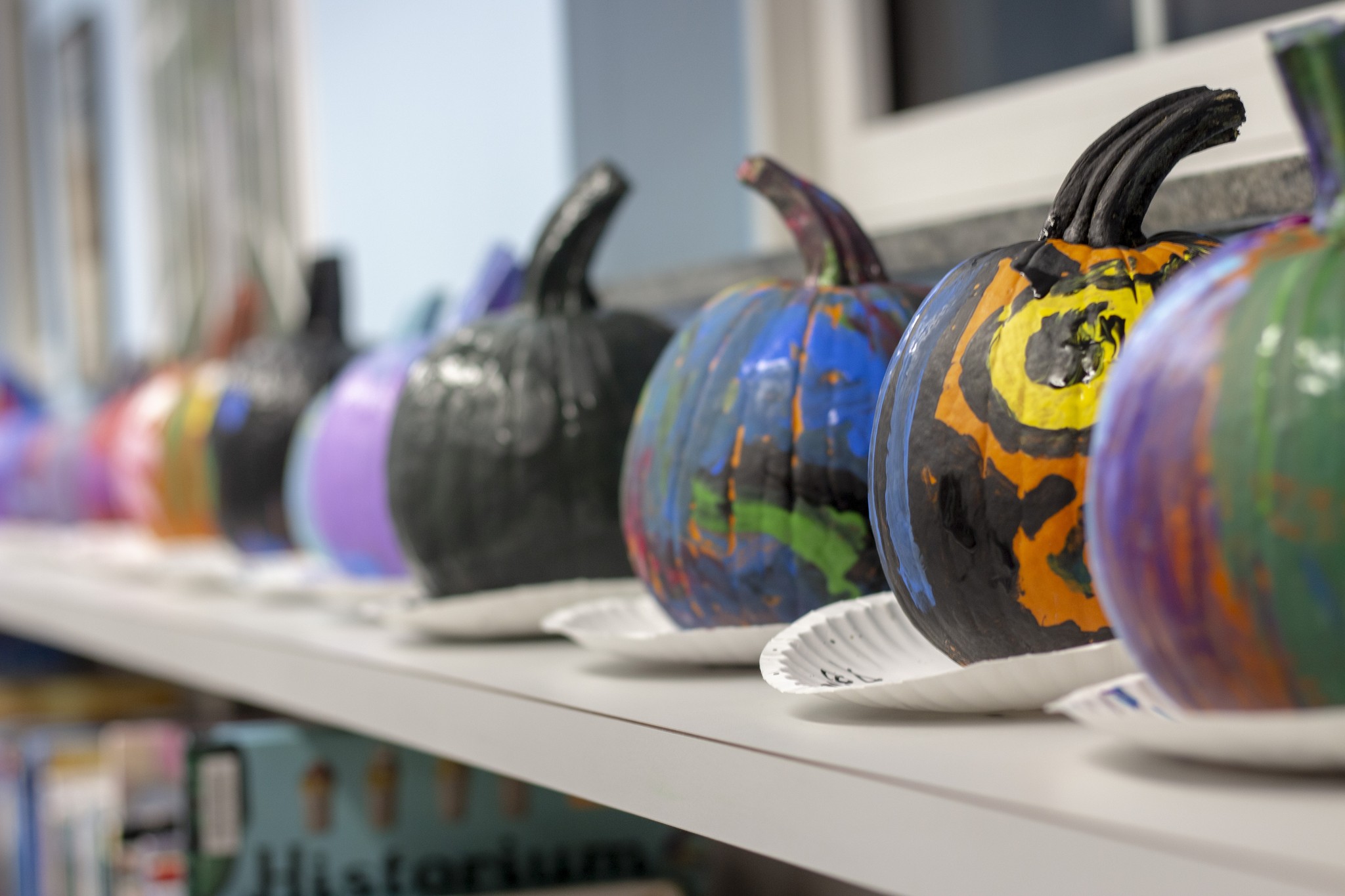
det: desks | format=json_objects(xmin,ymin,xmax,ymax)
[{"xmin": 1, "ymin": 513, "xmax": 1343, "ymax": 895}]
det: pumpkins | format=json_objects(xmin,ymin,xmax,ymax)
[{"xmin": 0, "ymin": 14, "xmax": 1345, "ymax": 714}]
